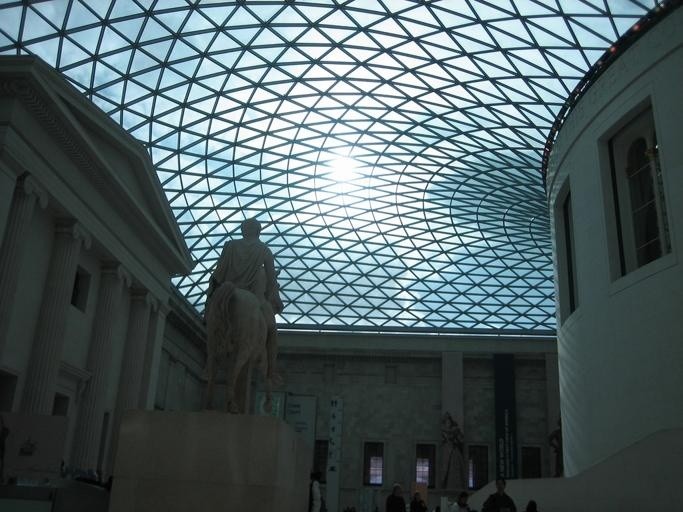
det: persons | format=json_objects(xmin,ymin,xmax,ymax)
[
  {"xmin": 479, "ymin": 476, "xmax": 516, "ymax": 511},
  {"xmin": 409, "ymin": 491, "xmax": 428, "ymax": 512},
  {"xmin": 384, "ymin": 483, "xmax": 407, "ymax": 511},
  {"xmin": 194, "ymin": 219, "xmax": 287, "ymax": 387},
  {"xmin": 449, "ymin": 491, "xmax": 470, "ymax": 511}
]
